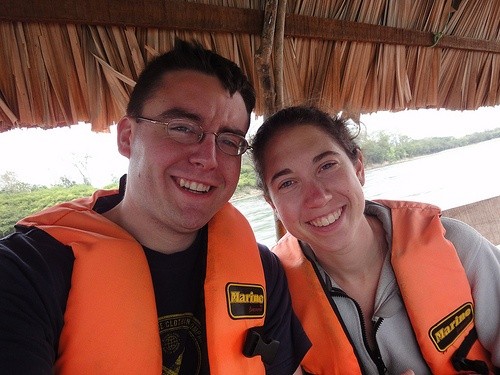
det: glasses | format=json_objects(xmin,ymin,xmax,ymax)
[{"xmin": 132, "ymin": 114, "xmax": 254, "ymax": 157}]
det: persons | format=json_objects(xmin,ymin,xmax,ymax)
[
  {"xmin": 0, "ymin": 36, "xmax": 313, "ymax": 375},
  {"xmin": 247, "ymin": 96, "xmax": 500, "ymax": 375}
]
